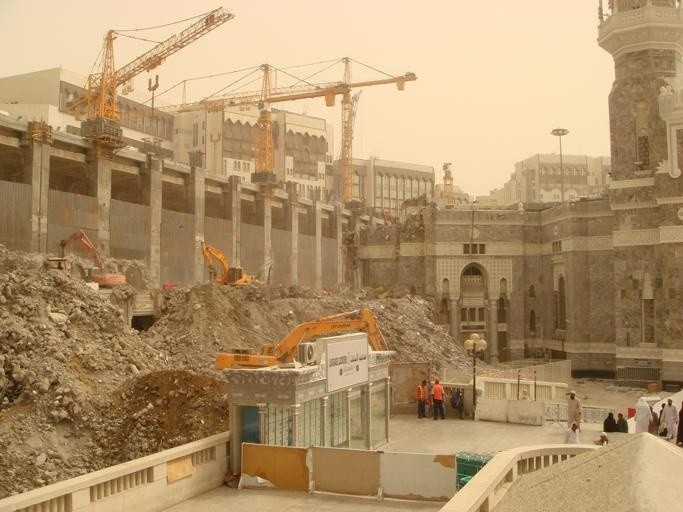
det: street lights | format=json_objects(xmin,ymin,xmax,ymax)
[
  {"xmin": 463, "ymin": 332, "xmax": 487, "ymax": 420},
  {"xmin": 552, "ymin": 128, "xmax": 570, "ymax": 203}
]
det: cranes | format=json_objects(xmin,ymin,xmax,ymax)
[
  {"xmin": 152, "ymin": 57, "xmax": 418, "ymax": 202},
  {"xmin": 65, "ymin": 6, "xmax": 236, "ymax": 149}
]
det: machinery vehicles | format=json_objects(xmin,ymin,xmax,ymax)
[
  {"xmin": 200, "ymin": 241, "xmax": 259, "ymax": 289},
  {"xmin": 215, "ymin": 309, "xmax": 396, "ymax": 371},
  {"xmin": 47, "ymin": 229, "xmax": 126, "ymax": 288}
]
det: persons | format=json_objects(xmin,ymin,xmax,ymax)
[
  {"xmin": 648, "ymin": 398, "xmax": 683, "ymax": 446},
  {"xmin": 567, "ymin": 391, "xmax": 582, "ymax": 433},
  {"xmin": 592, "ymin": 431, "xmax": 609, "ymax": 447},
  {"xmin": 449, "ymin": 390, "xmax": 465, "ymax": 420},
  {"xmin": 415, "ymin": 379, "xmax": 429, "ymax": 419},
  {"xmin": 616, "ymin": 412, "xmax": 628, "ymax": 433},
  {"xmin": 562, "ymin": 422, "xmax": 578, "ymax": 444},
  {"xmin": 432, "ymin": 377, "xmax": 446, "ymax": 420},
  {"xmin": 603, "ymin": 411, "xmax": 616, "ymax": 432}
]
